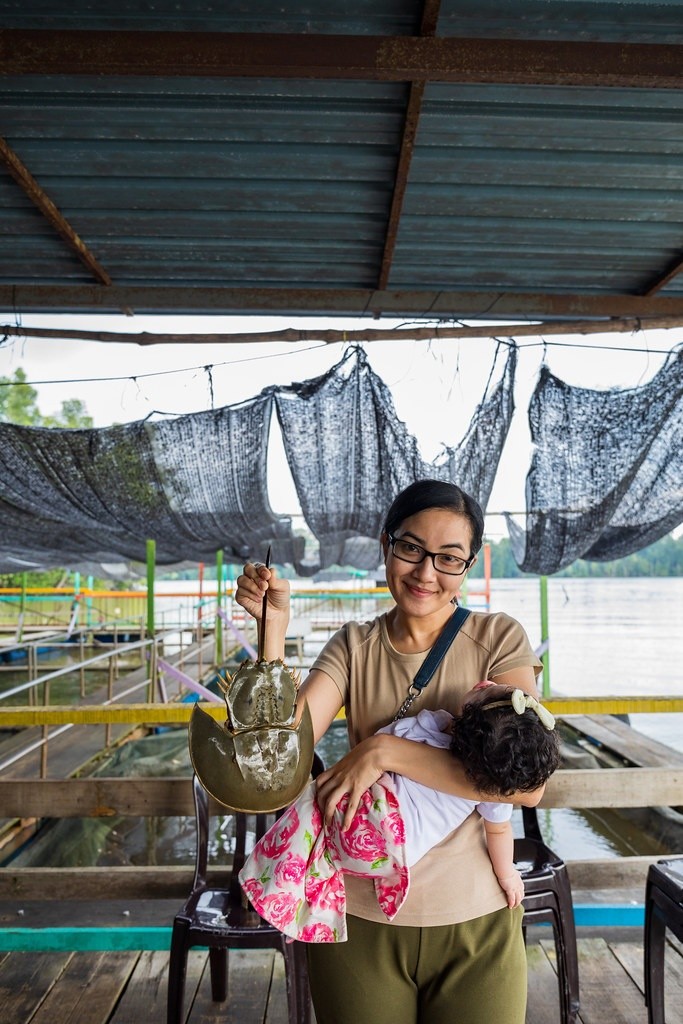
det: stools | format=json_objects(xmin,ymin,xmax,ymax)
[{"xmin": 642, "ymin": 858, "xmax": 683, "ymax": 1023}]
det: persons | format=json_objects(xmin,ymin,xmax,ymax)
[
  {"xmin": 234, "ymin": 481, "xmax": 550, "ymax": 1024},
  {"xmin": 237, "ymin": 678, "xmax": 562, "ymax": 948}
]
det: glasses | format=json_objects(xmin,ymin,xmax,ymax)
[{"xmin": 383, "ymin": 528, "xmax": 474, "ymax": 576}]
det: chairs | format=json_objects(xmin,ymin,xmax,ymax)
[
  {"xmin": 511, "ymin": 805, "xmax": 584, "ymax": 1024},
  {"xmin": 165, "ymin": 750, "xmax": 326, "ymax": 1024}
]
